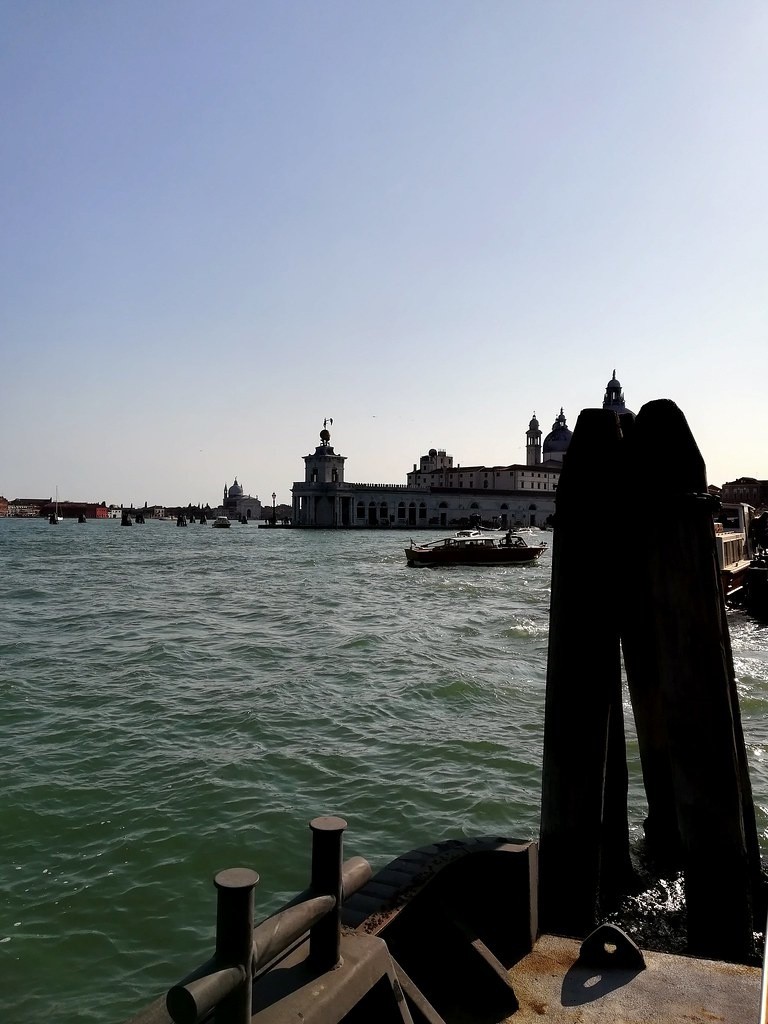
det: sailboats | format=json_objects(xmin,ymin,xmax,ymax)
[{"xmin": 44, "ymin": 486, "xmax": 64, "ymax": 521}]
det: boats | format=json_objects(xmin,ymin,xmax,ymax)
[
  {"xmin": 214, "ymin": 516, "xmax": 231, "ymax": 528},
  {"xmin": 404, "ymin": 529, "xmax": 550, "ymax": 567}
]
